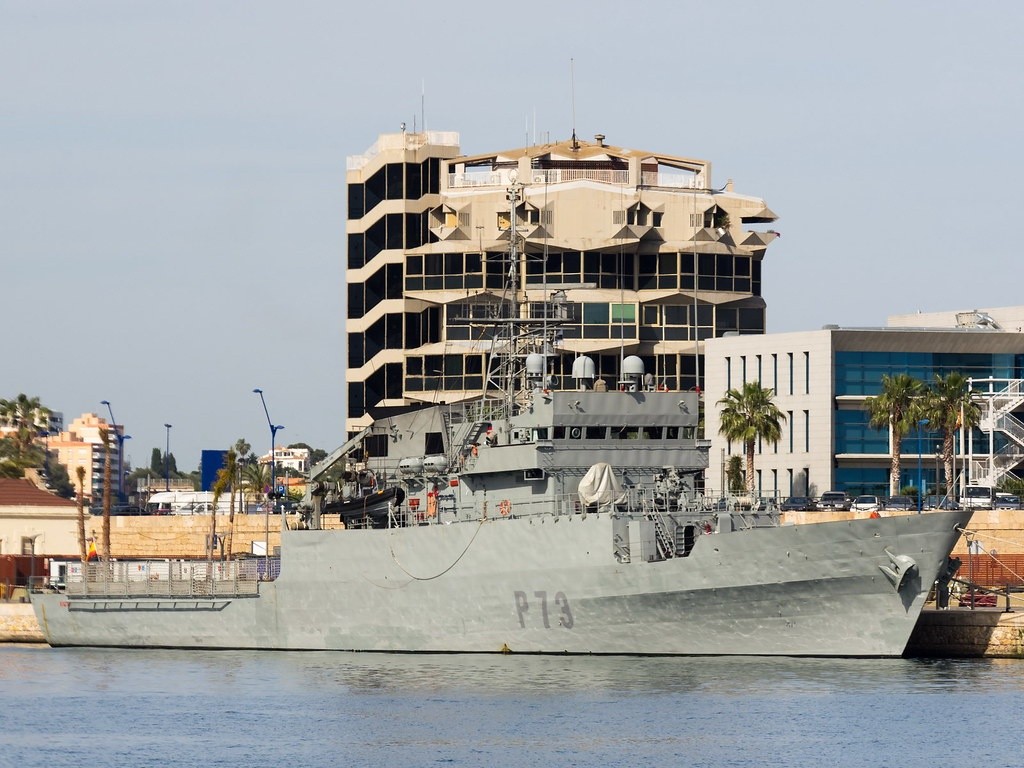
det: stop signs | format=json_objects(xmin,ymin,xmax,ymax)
[{"xmin": 265, "ymin": 484, "xmax": 270, "ymax": 492}]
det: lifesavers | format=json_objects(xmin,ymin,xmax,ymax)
[{"xmin": 499, "ymin": 499, "xmax": 512, "ymax": 516}]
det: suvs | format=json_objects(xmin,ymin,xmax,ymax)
[{"xmin": 816, "ymin": 490, "xmax": 851, "ymax": 511}]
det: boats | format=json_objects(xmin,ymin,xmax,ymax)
[
  {"xmin": 323, "ymin": 486, "xmax": 406, "ymax": 520},
  {"xmin": 24, "ymin": 180, "xmax": 976, "ymax": 657}
]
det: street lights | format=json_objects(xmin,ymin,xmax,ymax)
[
  {"xmin": 39, "ymin": 431, "xmax": 50, "ymax": 477},
  {"xmin": 99, "ymin": 400, "xmax": 132, "ymax": 505},
  {"xmin": 253, "ymin": 389, "xmax": 285, "ymax": 498},
  {"xmin": 163, "ymin": 423, "xmax": 172, "ymax": 492}
]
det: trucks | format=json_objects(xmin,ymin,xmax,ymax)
[{"xmin": 959, "ymin": 483, "xmax": 1003, "ymax": 510}]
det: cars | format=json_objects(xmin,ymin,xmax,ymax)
[
  {"xmin": 992, "ymin": 493, "xmax": 1024, "ymax": 510},
  {"xmin": 780, "ymin": 496, "xmax": 818, "ymax": 511},
  {"xmin": 923, "ymin": 495, "xmax": 959, "ymax": 511},
  {"xmin": 884, "ymin": 495, "xmax": 916, "ymax": 511},
  {"xmin": 176, "ymin": 502, "xmax": 224, "ymax": 514},
  {"xmin": 97, "ymin": 506, "xmax": 150, "ymax": 515},
  {"xmin": 271, "ymin": 501, "xmax": 301, "ymax": 514},
  {"xmin": 849, "ymin": 494, "xmax": 886, "ymax": 512},
  {"xmin": 148, "ymin": 509, "xmax": 176, "ymax": 515},
  {"xmin": 710, "ymin": 497, "xmax": 778, "ymax": 514}
]
